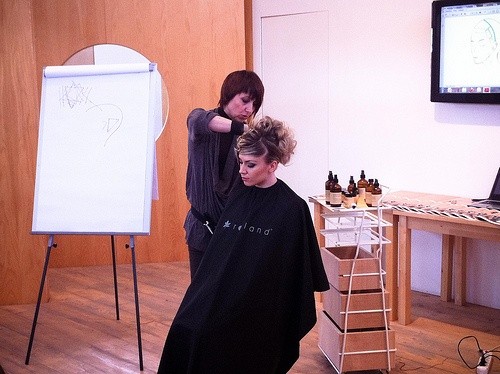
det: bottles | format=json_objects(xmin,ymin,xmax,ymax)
[
  {"xmin": 351, "ymin": 183, "xmax": 359, "ymax": 208},
  {"xmin": 357, "ymin": 170, "xmax": 369, "ymax": 201},
  {"xmin": 330, "ymin": 175, "xmax": 342, "ymax": 207},
  {"xmin": 365, "ymin": 178, "xmax": 374, "ymax": 207},
  {"xmin": 325, "ymin": 170, "xmax": 334, "ymax": 205},
  {"xmin": 341, "ymin": 188, "xmax": 352, "ymax": 208},
  {"xmin": 371, "ymin": 179, "xmax": 382, "ymax": 207},
  {"xmin": 348, "ymin": 176, "xmax": 355, "ymax": 193}
]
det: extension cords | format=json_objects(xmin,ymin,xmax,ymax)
[{"xmin": 476, "ymin": 350, "xmax": 492, "ymax": 374}]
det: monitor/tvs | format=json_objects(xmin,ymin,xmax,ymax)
[{"xmin": 430, "ymin": 0, "xmax": 500, "ymax": 104}]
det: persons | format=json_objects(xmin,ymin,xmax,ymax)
[
  {"xmin": 182, "ymin": 70, "xmax": 264, "ymax": 283},
  {"xmin": 157, "ymin": 116, "xmax": 330, "ymax": 374}
]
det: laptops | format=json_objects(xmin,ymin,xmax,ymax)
[{"xmin": 467, "ymin": 167, "xmax": 500, "ymax": 210}]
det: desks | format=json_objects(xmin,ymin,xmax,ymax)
[
  {"xmin": 395, "ymin": 195, "xmax": 500, "ymax": 326},
  {"xmin": 308, "ymin": 192, "xmax": 397, "ymax": 322}
]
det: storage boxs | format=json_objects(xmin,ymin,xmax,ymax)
[
  {"xmin": 322, "ymin": 283, "xmax": 390, "ymax": 330},
  {"xmin": 318, "ymin": 310, "xmax": 396, "ymax": 372},
  {"xmin": 320, "ymin": 245, "xmax": 383, "ymax": 292}
]
